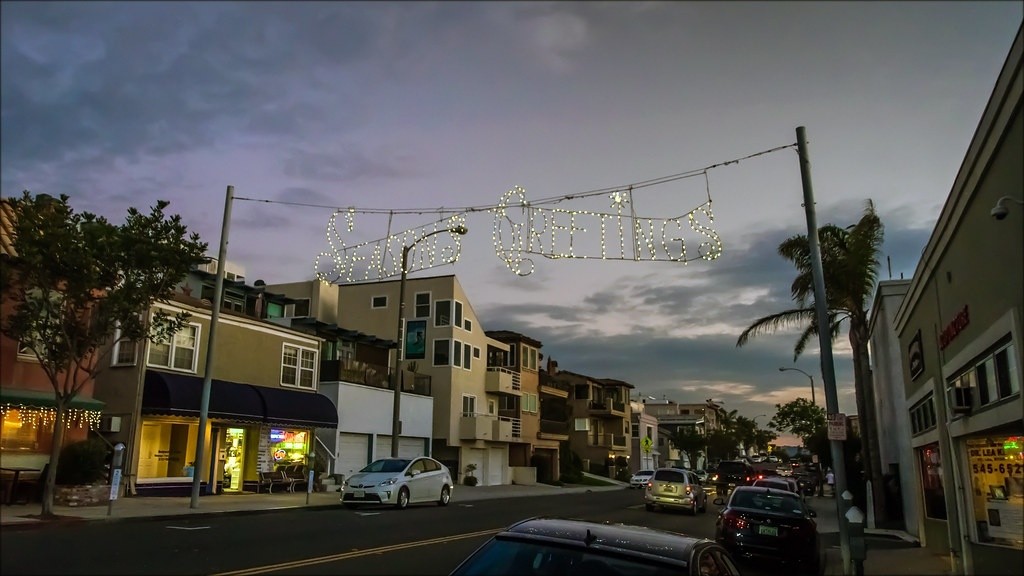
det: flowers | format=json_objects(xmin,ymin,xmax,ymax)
[{"xmin": 187, "ymin": 461, "xmax": 194, "ymax": 467}]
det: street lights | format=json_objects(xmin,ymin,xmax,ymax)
[
  {"xmin": 753, "ymin": 414, "xmax": 766, "ymax": 454},
  {"xmin": 704, "ymin": 401, "xmax": 724, "ymax": 469},
  {"xmin": 779, "ymin": 367, "xmax": 816, "ymax": 408},
  {"xmin": 391, "ymin": 227, "xmax": 469, "ymax": 457}
]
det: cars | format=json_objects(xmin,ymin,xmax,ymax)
[
  {"xmin": 714, "ymin": 486, "xmax": 820, "ymax": 575},
  {"xmin": 630, "ymin": 469, "xmax": 655, "ymax": 489},
  {"xmin": 751, "ymin": 454, "xmax": 817, "ymax": 507},
  {"xmin": 693, "ymin": 470, "xmax": 709, "ymax": 485},
  {"xmin": 645, "ymin": 467, "xmax": 708, "ymax": 515},
  {"xmin": 342, "ymin": 456, "xmax": 454, "ymax": 509},
  {"xmin": 706, "ymin": 468, "xmax": 718, "ymax": 481},
  {"xmin": 444, "ymin": 514, "xmax": 746, "ymax": 576}
]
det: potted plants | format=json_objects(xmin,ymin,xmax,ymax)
[{"xmin": 464, "ymin": 464, "xmax": 478, "ymax": 487}]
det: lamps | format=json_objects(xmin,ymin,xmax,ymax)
[{"xmin": 991, "ymin": 196, "xmax": 1024, "ymax": 220}]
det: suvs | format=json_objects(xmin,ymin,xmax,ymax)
[{"xmin": 714, "ymin": 461, "xmax": 752, "ymax": 494}]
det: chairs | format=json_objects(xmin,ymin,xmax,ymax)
[
  {"xmin": 295, "ymin": 465, "xmax": 304, "ymax": 471},
  {"xmin": 276, "ymin": 466, "xmax": 286, "ymax": 472},
  {"xmin": 286, "ymin": 465, "xmax": 296, "ymax": 471}
]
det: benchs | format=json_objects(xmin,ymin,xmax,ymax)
[
  {"xmin": 258, "ymin": 471, "xmax": 292, "ymax": 495},
  {"xmin": 284, "ymin": 471, "xmax": 307, "ymax": 493}
]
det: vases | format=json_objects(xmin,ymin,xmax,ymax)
[{"xmin": 186, "ymin": 468, "xmax": 194, "ymax": 476}]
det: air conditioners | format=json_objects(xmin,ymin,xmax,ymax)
[{"xmin": 947, "ymin": 387, "xmax": 970, "ymax": 413}]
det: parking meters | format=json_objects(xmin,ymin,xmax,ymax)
[
  {"xmin": 306, "ymin": 452, "xmax": 317, "ymax": 507},
  {"xmin": 109, "ymin": 445, "xmax": 125, "ymax": 515}
]
